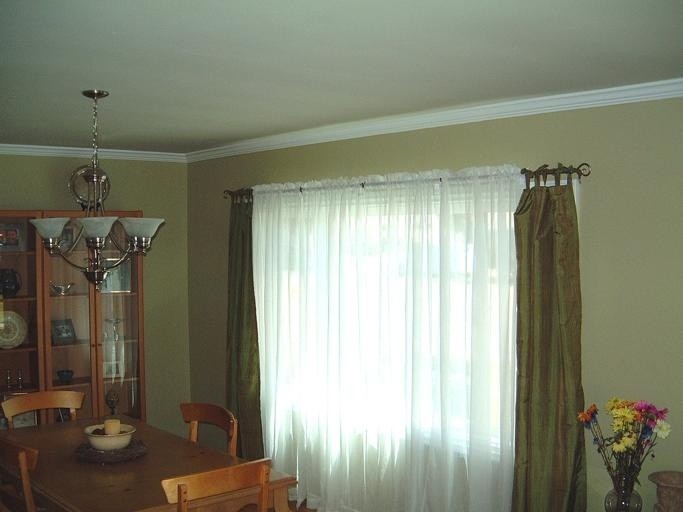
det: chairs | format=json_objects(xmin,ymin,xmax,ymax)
[
  {"xmin": 0, "ymin": 388, "xmax": 86, "ymax": 436},
  {"xmin": 0, "ymin": 439, "xmax": 42, "ymax": 512},
  {"xmin": 180, "ymin": 402, "xmax": 238, "ymax": 459},
  {"xmin": 162, "ymin": 458, "xmax": 272, "ymax": 512}
]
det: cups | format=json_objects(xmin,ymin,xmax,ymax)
[
  {"xmin": 4, "ymin": 368, "xmax": 24, "ymax": 389},
  {"xmin": 56, "ymin": 371, "xmax": 73, "ymax": 383}
]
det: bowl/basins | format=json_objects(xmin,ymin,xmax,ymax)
[{"xmin": 84, "ymin": 424, "xmax": 135, "ymax": 451}]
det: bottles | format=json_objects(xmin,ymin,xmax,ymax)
[{"xmin": 0, "ymin": 223, "xmax": 24, "ymax": 250}]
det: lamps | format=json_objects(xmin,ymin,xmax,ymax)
[{"xmin": 28, "ymin": 89, "xmax": 166, "ymax": 288}]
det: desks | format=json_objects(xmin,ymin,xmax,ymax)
[{"xmin": 1, "ymin": 416, "xmax": 298, "ymax": 512}]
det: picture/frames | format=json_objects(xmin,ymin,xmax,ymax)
[
  {"xmin": 52, "ymin": 319, "xmax": 76, "ymax": 346},
  {"xmin": 4, "ymin": 393, "xmax": 37, "ymax": 428}
]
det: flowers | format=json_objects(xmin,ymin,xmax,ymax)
[{"xmin": 579, "ymin": 398, "xmax": 673, "ymax": 478}]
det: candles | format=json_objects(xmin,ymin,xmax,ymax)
[{"xmin": 104, "ymin": 420, "xmax": 121, "ymax": 435}]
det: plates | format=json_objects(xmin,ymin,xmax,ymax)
[{"xmin": 0, "ymin": 311, "xmax": 28, "ymax": 350}]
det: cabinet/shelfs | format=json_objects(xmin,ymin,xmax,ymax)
[
  {"xmin": 0, "ymin": 210, "xmax": 45, "ymax": 428},
  {"xmin": 44, "ymin": 209, "xmax": 146, "ymax": 426}
]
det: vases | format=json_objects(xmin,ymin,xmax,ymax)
[
  {"xmin": 602, "ymin": 471, "xmax": 642, "ymax": 511},
  {"xmin": 649, "ymin": 471, "xmax": 682, "ymax": 511}
]
